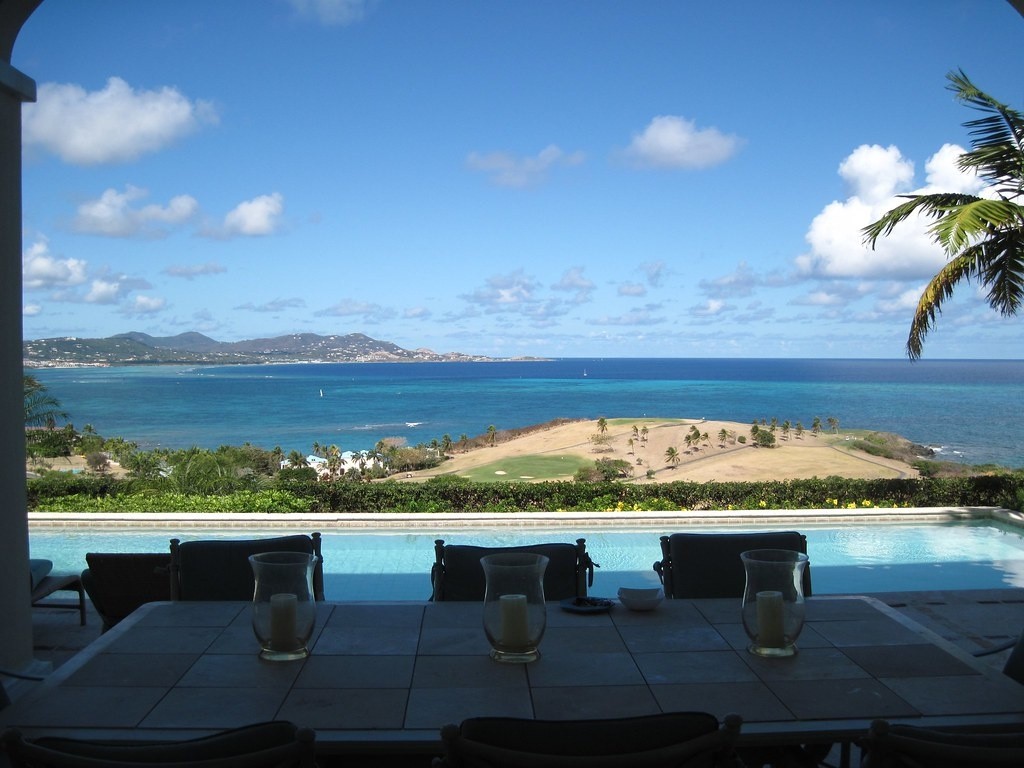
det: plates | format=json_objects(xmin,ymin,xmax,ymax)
[{"xmin": 559, "ymin": 596, "xmax": 616, "ymax": 613}]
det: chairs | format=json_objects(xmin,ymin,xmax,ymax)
[
  {"xmin": 431, "ymin": 710, "xmax": 743, "ymax": 768},
  {"xmin": 1, "ymin": 716, "xmax": 316, "ymax": 768},
  {"xmin": 851, "ymin": 720, "xmax": 1024, "ymax": 768},
  {"xmin": 80, "ymin": 530, "xmax": 324, "ymax": 636},
  {"xmin": 428, "ymin": 539, "xmax": 594, "ymax": 603},
  {"xmin": 652, "ymin": 529, "xmax": 814, "ymax": 598}
]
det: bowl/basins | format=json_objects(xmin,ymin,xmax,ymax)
[{"xmin": 618, "ymin": 587, "xmax": 666, "ymax": 612}]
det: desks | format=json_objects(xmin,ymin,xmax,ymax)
[{"xmin": 0, "ymin": 589, "xmax": 1024, "ymax": 768}]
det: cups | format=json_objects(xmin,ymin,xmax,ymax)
[
  {"xmin": 739, "ymin": 549, "xmax": 811, "ymax": 662},
  {"xmin": 246, "ymin": 551, "xmax": 318, "ymax": 661},
  {"xmin": 479, "ymin": 552, "xmax": 550, "ymax": 664}
]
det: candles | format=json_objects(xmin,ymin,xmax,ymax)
[
  {"xmin": 756, "ymin": 588, "xmax": 785, "ymax": 646},
  {"xmin": 269, "ymin": 592, "xmax": 299, "ymax": 646},
  {"xmin": 498, "ymin": 588, "xmax": 531, "ymax": 647}
]
